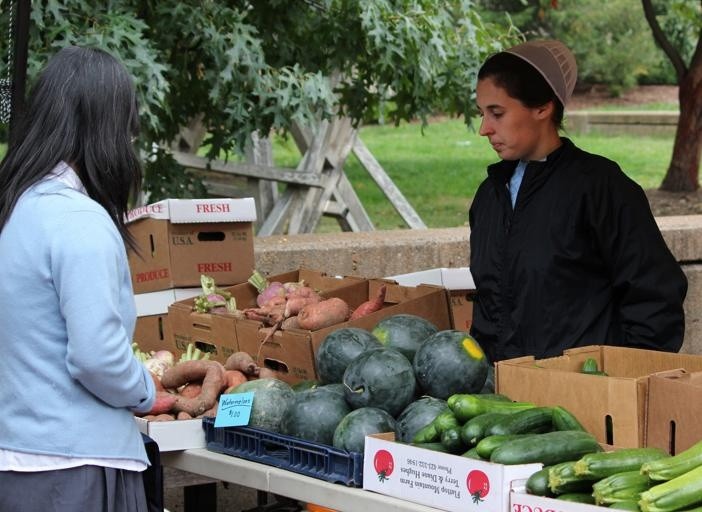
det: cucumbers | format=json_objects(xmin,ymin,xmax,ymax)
[{"xmin": 408, "ymin": 391, "xmax": 702, "ymax": 512}]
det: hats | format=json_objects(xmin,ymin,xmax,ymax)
[{"xmin": 502, "ymin": 40, "xmax": 578, "ymax": 108}]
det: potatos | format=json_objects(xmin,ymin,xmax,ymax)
[{"xmin": 147, "ymin": 285, "xmax": 386, "ymax": 429}]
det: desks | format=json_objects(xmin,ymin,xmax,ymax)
[{"xmin": 154, "ymin": 449, "xmax": 447, "ymax": 512}]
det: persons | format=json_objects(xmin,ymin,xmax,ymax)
[
  {"xmin": 468, "ymin": 40, "xmax": 688, "ymax": 361},
  {"xmin": 0, "ymin": 46, "xmax": 156, "ymax": 512}
]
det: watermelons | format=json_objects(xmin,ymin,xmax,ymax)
[{"xmin": 227, "ymin": 312, "xmax": 489, "ymax": 454}]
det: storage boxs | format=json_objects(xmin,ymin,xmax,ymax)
[
  {"xmin": 493, "ymin": 345, "xmax": 702, "ymax": 456},
  {"xmin": 363, "ymin": 432, "xmax": 630, "ymax": 512},
  {"xmin": 122, "ymin": 196, "xmax": 258, "ymax": 371},
  {"xmin": 168, "ymin": 267, "xmax": 474, "ymax": 384}
]
